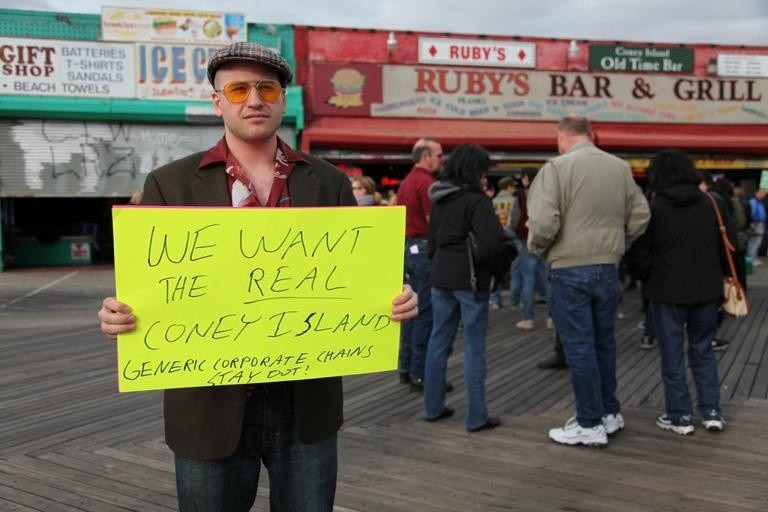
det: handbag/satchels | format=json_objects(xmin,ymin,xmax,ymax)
[{"xmin": 720, "ymin": 279, "xmax": 748, "ymax": 317}]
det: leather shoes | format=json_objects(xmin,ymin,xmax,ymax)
[
  {"xmin": 538, "ymin": 357, "xmax": 566, "ymax": 369},
  {"xmin": 426, "ymin": 407, "xmax": 454, "ymax": 422},
  {"xmin": 467, "ymin": 415, "xmax": 501, "ymax": 431}
]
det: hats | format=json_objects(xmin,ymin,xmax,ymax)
[{"xmin": 207, "ymin": 42, "xmax": 293, "ymax": 87}]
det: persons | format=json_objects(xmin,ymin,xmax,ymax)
[
  {"xmin": 99, "ymin": 36, "xmax": 420, "ymax": 512},
  {"xmin": 340, "ymin": 110, "xmax": 768, "ymax": 446}
]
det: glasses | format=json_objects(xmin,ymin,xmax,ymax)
[{"xmin": 217, "ymin": 80, "xmax": 283, "ymax": 105}]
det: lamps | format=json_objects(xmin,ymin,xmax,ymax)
[
  {"xmin": 563, "ymin": 39, "xmax": 582, "ymax": 70},
  {"xmin": 384, "ymin": 32, "xmax": 398, "ymax": 63},
  {"xmin": 698, "ymin": 56, "xmax": 720, "ymax": 74}
]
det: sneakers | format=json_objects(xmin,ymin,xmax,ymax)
[
  {"xmin": 700, "ymin": 407, "xmax": 727, "ymax": 432},
  {"xmin": 399, "ymin": 370, "xmax": 452, "ymax": 392},
  {"xmin": 711, "ymin": 339, "xmax": 731, "ymax": 352},
  {"xmin": 517, "ymin": 321, "xmax": 536, "ymax": 331},
  {"xmin": 602, "ymin": 411, "xmax": 624, "ymax": 434},
  {"xmin": 657, "ymin": 412, "xmax": 694, "ymax": 434},
  {"xmin": 641, "ymin": 335, "xmax": 654, "ymax": 348},
  {"xmin": 548, "ymin": 419, "xmax": 608, "ymax": 446},
  {"xmin": 489, "ymin": 293, "xmax": 545, "ymax": 310}
]
